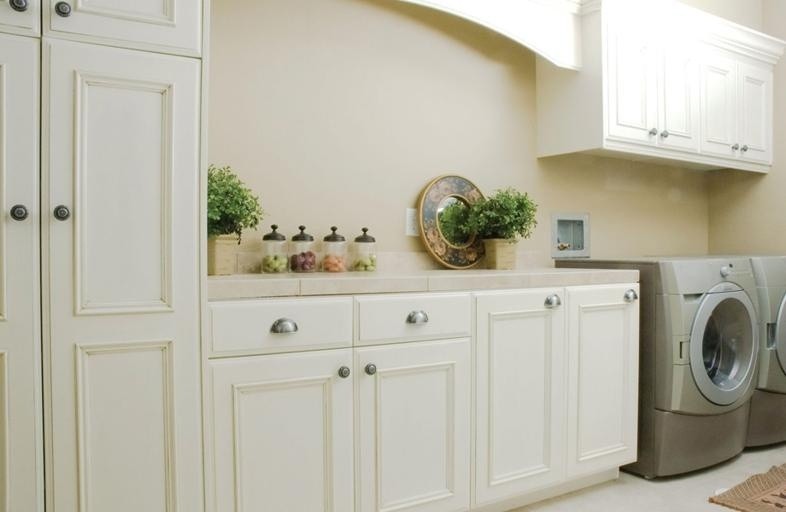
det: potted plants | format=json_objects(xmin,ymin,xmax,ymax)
[
  {"xmin": 206, "ymin": 161, "xmax": 267, "ymax": 277},
  {"xmin": 456, "ymin": 185, "xmax": 539, "ymax": 271}
]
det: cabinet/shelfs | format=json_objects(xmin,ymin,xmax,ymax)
[
  {"xmin": 208, "ymin": 281, "xmax": 644, "ymax": 512},
  {"xmin": 531, "ymin": 1, "xmax": 785, "ymax": 175},
  {"xmin": 1, "ymin": 1, "xmax": 211, "ymax": 511}
]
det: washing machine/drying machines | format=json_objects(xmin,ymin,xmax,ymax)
[
  {"xmin": 551, "ymin": 256, "xmax": 761, "ymax": 480},
  {"xmin": 743, "ymin": 256, "xmax": 785, "ymax": 448}
]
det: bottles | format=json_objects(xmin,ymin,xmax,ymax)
[
  {"xmin": 287, "ymin": 224, "xmax": 317, "ymax": 272},
  {"xmin": 322, "ymin": 226, "xmax": 347, "ymax": 273},
  {"xmin": 260, "ymin": 224, "xmax": 289, "ymax": 273},
  {"xmin": 353, "ymin": 224, "xmax": 379, "ymax": 272}
]
